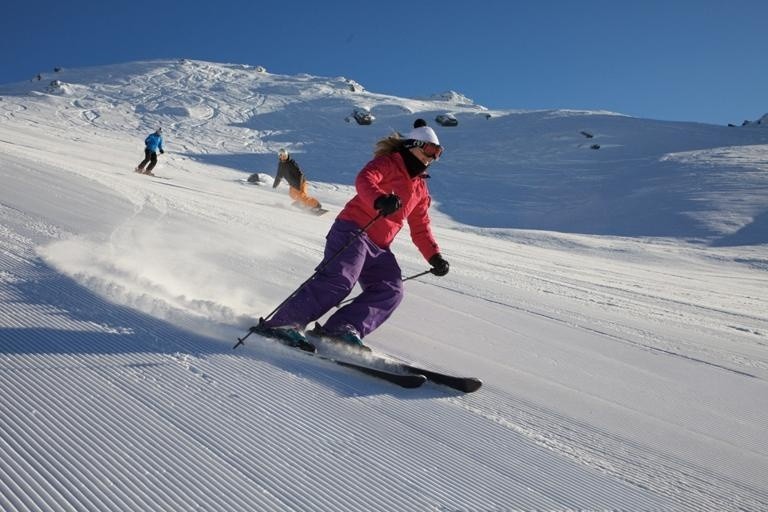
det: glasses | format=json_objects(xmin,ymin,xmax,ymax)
[{"xmin": 404, "ymin": 139, "xmax": 444, "ymax": 160}]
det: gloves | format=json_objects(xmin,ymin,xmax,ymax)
[
  {"xmin": 145, "ymin": 142, "xmax": 148, "ymax": 145},
  {"xmin": 374, "ymin": 193, "xmax": 402, "ymax": 215},
  {"xmin": 428, "ymin": 253, "xmax": 449, "ymax": 275},
  {"xmin": 160, "ymin": 149, "xmax": 163, "ymax": 153}
]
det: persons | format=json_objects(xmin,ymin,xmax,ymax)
[
  {"xmin": 137, "ymin": 127, "xmax": 165, "ymax": 175},
  {"xmin": 251, "ymin": 117, "xmax": 451, "ymax": 354},
  {"xmin": 271, "ymin": 147, "xmax": 323, "ymax": 208}
]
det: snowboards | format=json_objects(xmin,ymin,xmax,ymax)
[{"xmin": 292, "ymin": 201, "xmax": 329, "ymax": 216}]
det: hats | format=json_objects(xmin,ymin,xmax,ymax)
[
  {"xmin": 403, "ymin": 118, "xmax": 440, "ymax": 146},
  {"xmin": 156, "ymin": 127, "xmax": 163, "ymax": 132},
  {"xmin": 278, "ymin": 149, "xmax": 288, "ymax": 155}
]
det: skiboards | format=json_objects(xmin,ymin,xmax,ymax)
[
  {"xmin": 132, "ymin": 168, "xmax": 172, "ymax": 179},
  {"xmin": 249, "ymin": 318, "xmax": 482, "ymax": 393}
]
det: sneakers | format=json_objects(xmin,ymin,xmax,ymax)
[
  {"xmin": 274, "ymin": 328, "xmax": 316, "ymax": 353},
  {"xmin": 315, "ymin": 203, "xmax": 321, "ymax": 213},
  {"xmin": 138, "ymin": 168, "xmax": 154, "ymax": 176},
  {"xmin": 340, "ymin": 330, "xmax": 371, "ymax": 352}
]
